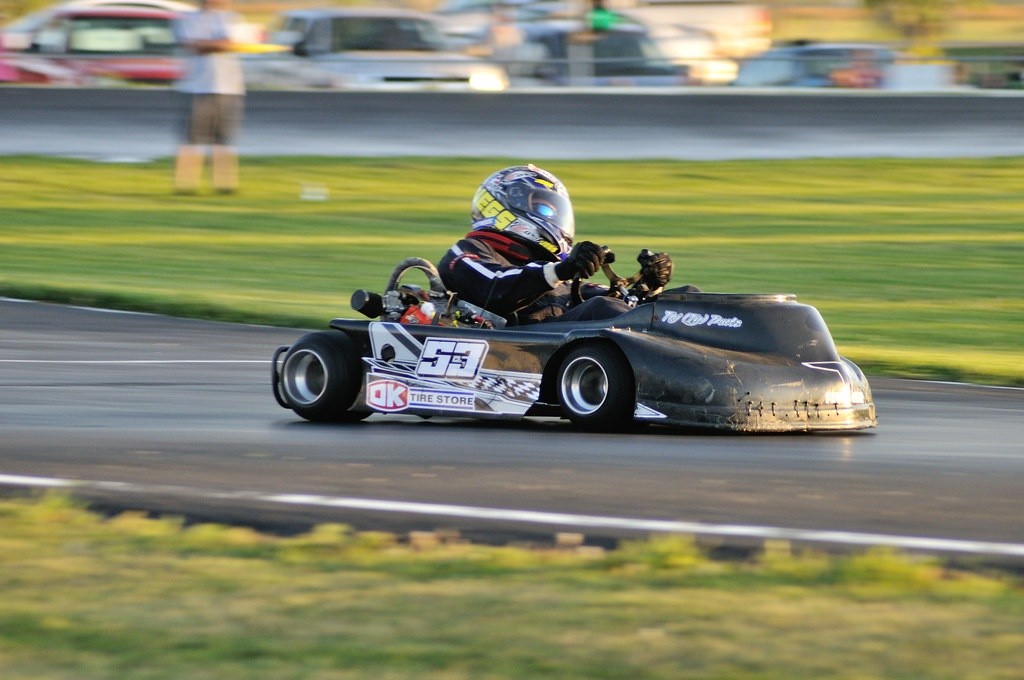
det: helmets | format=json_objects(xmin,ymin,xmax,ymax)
[{"xmin": 471, "ymin": 164, "xmax": 575, "ymax": 259}]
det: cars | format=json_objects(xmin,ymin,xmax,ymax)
[
  {"xmin": 441, "ymin": 2, "xmax": 694, "ymax": 87},
  {"xmin": 0, "ymin": 3, "xmax": 266, "ymax": 84},
  {"xmin": 727, "ymin": 35, "xmax": 960, "ymax": 88},
  {"xmin": 278, "ymin": 11, "xmax": 512, "ymax": 93}
]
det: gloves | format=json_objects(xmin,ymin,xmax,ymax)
[
  {"xmin": 554, "ymin": 241, "xmax": 606, "ymax": 281},
  {"xmin": 637, "ymin": 249, "xmax": 672, "ymax": 293}
]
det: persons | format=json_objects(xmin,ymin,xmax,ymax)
[
  {"xmin": 437, "ymin": 164, "xmax": 672, "ymax": 328},
  {"xmin": 176, "ymin": 0, "xmax": 246, "ymax": 196}
]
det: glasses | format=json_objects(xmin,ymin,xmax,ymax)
[{"xmin": 534, "ymin": 203, "xmax": 563, "ymax": 223}]
